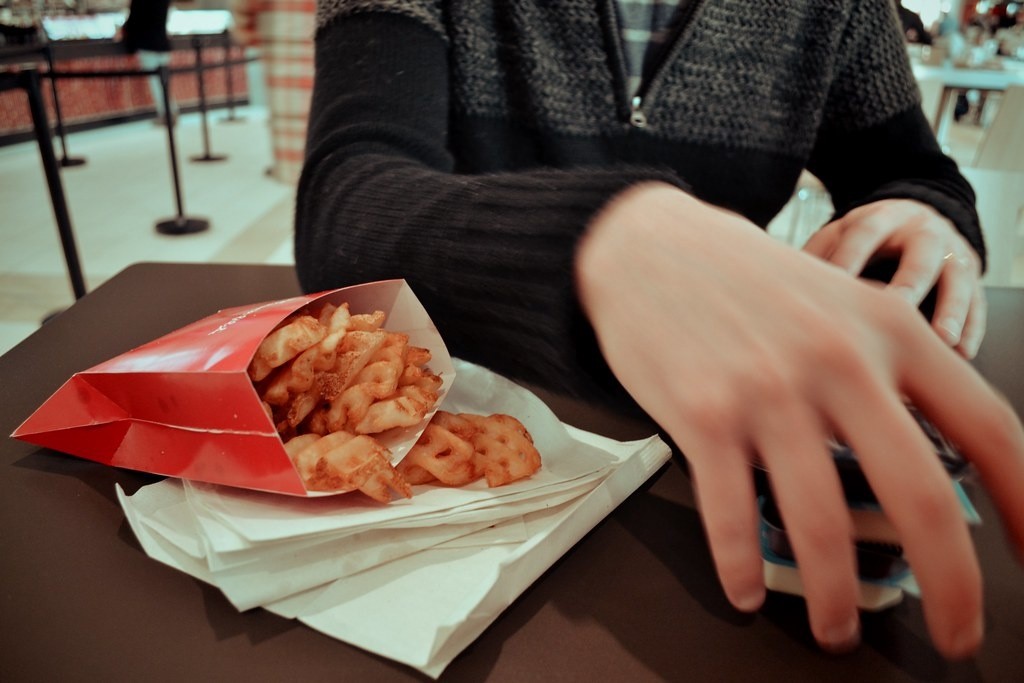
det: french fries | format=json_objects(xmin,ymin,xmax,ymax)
[{"xmin": 249, "ymin": 303, "xmax": 443, "ymax": 502}]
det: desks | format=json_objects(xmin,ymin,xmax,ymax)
[
  {"xmin": 0, "ymin": 264, "xmax": 1024, "ymax": 683},
  {"xmin": 909, "ymin": 43, "xmax": 1024, "ymax": 142}
]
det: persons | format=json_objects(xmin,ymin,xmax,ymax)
[
  {"xmin": 895, "ymin": 0, "xmax": 1024, "ymax": 124},
  {"xmin": 113, "ymin": 0, "xmax": 181, "ymax": 127},
  {"xmin": 291, "ymin": 0, "xmax": 1024, "ymax": 658}
]
